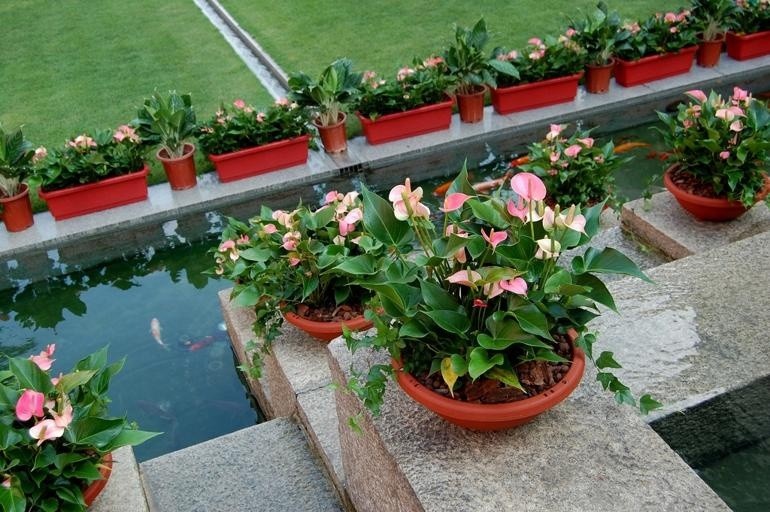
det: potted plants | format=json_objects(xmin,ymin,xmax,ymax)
[
  {"xmin": 688, "ymin": 1, "xmax": 740, "ymax": 69},
  {"xmin": 612, "ymin": 8, "xmax": 699, "ymax": 89},
  {"xmin": 561, "ymin": 2, "xmax": 620, "ymax": 95},
  {"xmin": 0, "ymin": 120, "xmax": 43, "ymax": 232},
  {"xmin": 485, "ymin": 31, "xmax": 587, "ymax": 116},
  {"xmin": 354, "ymin": 53, "xmax": 458, "ymax": 146},
  {"xmin": 441, "ymin": 15, "xmax": 523, "ymax": 124},
  {"xmin": 192, "ymin": 99, "xmax": 312, "ymax": 183},
  {"xmin": 285, "ymin": 56, "xmax": 360, "ymax": 155},
  {"xmin": 27, "ymin": 125, "xmax": 155, "ymax": 223},
  {"xmin": 130, "ymin": 85, "xmax": 201, "ymax": 192},
  {"xmin": 727, "ymin": 1, "xmax": 769, "ymax": 61}
]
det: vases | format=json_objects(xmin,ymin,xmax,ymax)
[
  {"xmin": 73, "ymin": 444, "xmax": 114, "ymax": 506},
  {"xmin": 388, "ymin": 324, "xmax": 586, "ymax": 432},
  {"xmin": 278, "ymin": 295, "xmax": 383, "ymax": 340}
]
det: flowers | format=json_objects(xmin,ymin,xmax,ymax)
[
  {"xmin": 1, "ymin": 342, "xmax": 165, "ymax": 511},
  {"xmin": 329, "ymin": 119, "xmax": 675, "ymax": 439},
  {"xmin": 215, "ymin": 188, "xmax": 404, "ymax": 379}
]
